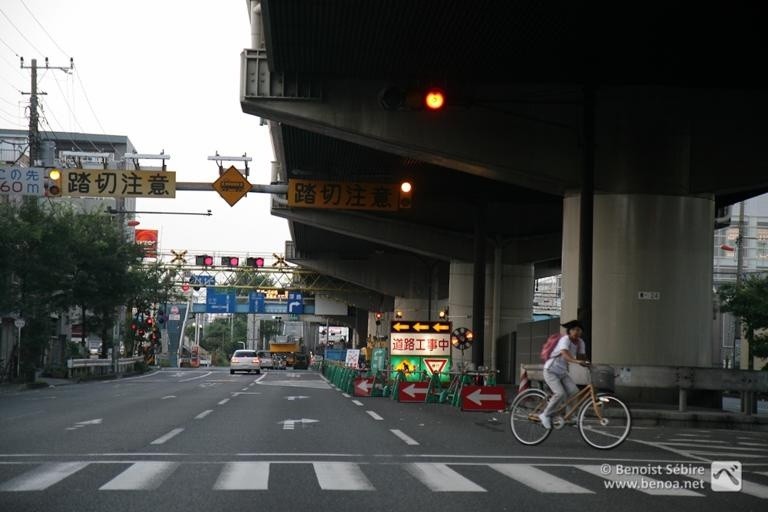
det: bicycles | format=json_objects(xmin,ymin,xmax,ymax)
[{"xmin": 508, "ymin": 360, "xmax": 633, "ymax": 450}]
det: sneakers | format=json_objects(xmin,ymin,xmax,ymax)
[{"xmin": 540, "ymin": 412, "xmax": 551, "ymax": 429}]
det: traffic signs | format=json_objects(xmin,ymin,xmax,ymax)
[
  {"xmin": 288, "ymin": 291, "xmax": 304, "ymax": 314},
  {"xmin": 248, "ymin": 291, "xmax": 265, "ymax": 313},
  {"xmin": 206, "ymin": 286, "xmax": 236, "ymax": 313}
]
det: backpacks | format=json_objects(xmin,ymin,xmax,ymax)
[{"xmin": 541, "ymin": 333, "xmax": 581, "ymax": 360}]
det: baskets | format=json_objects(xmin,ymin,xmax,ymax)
[{"xmin": 591, "ymin": 363, "xmax": 615, "ymax": 393}]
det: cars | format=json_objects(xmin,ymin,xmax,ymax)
[
  {"xmin": 230, "ymin": 350, "xmax": 260, "ymax": 374},
  {"xmin": 278, "ymin": 356, "xmax": 287, "ymax": 369},
  {"xmin": 257, "ymin": 350, "xmax": 279, "ymax": 370}
]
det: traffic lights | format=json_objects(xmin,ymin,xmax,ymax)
[
  {"xmin": 246, "ymin": 257, "xmax": 264, "ymax": 267},
  {"xmin": 152, "ymin": 327, "xmax": 158, "ymax": 340},
  {"xmin": 48, "ymin": 168, "xmax": 61, "ymax": 197},
  {"xmin": 157, "ymin": 309, "xmax": 164, "ymax": 323},
  {"xmin": 381, "ymin": 86, "xmax": 446, "ymax": 111},
  {"xmin": 222, "ymin": 256, "xmax": 239, "ymax": 266},
  {"xmin": 376, "ymin": 312, "xmax": 381, "ymax": 326},
  {"xmin": 145, "ymin": 309, "xmax": 152, "ymax": 325},
  {"xmin": 196, "ymin": 255, "xmax": 213, "ymax": 266},
  {"xmin": 399, "ymin": 182, "xmax": 412, "ymax": 209}
]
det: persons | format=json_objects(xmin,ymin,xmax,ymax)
[
  {"xmin": 271, "ymin": 353, "xmax": 283, "ymax": 370},
  {"xmin": 538, "ymin": 319, "xmax": 591, "ymax": 430}
]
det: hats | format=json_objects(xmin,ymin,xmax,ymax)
[{"xmin": 561, "ymin": 320, "xmax": 583, "ymax": 329}]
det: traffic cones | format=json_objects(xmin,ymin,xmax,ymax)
[{"xmin": 517, "ymin": 368, "xmax": 534, "ymax": 402}]
